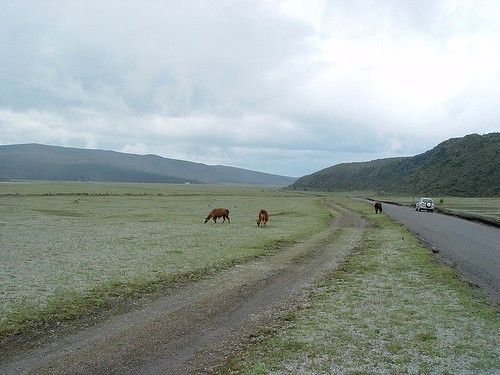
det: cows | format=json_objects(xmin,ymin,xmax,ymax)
[
  {"xmin": 204, "ymin": 207, "xmax": 231, "ymax": 224},
  {"xmin": 373, "ymin": 202, "xmax": 383, "ymax": 214},
  {"xmin": 255, "ymin": 209, "xmax": 269, "ymax": 228}
]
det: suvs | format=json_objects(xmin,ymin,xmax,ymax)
[{"xmin": 415, "ymin": 197, "xmax": 434, "ymax": 212}]
